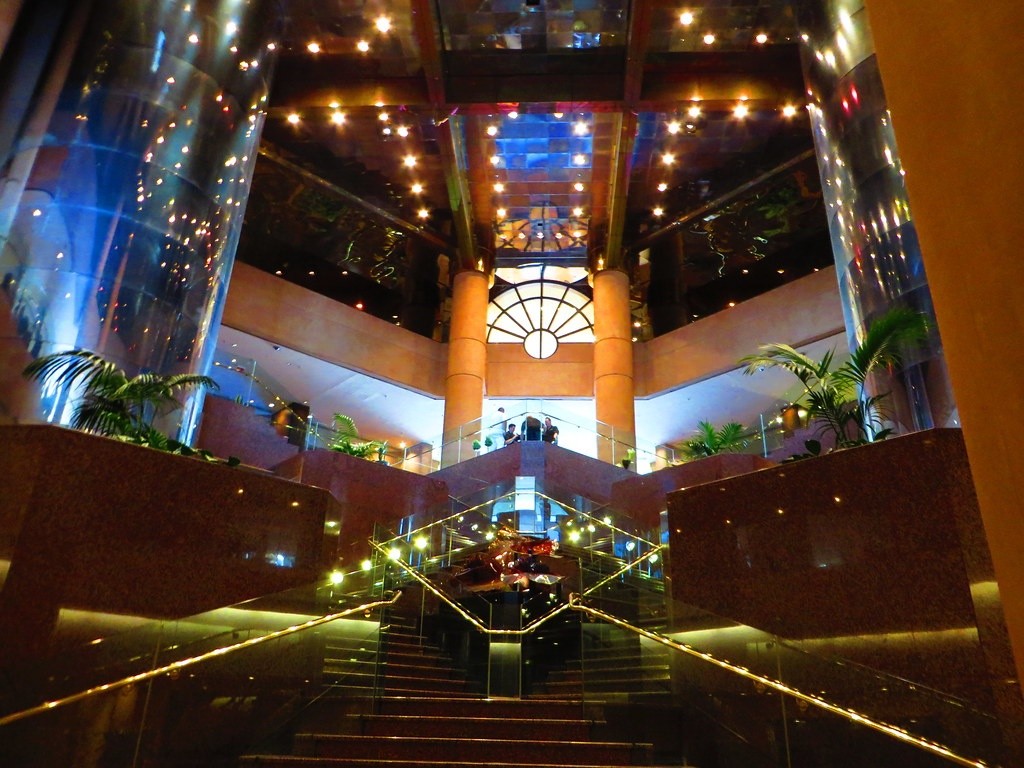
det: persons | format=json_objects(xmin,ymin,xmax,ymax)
[
  {"xmin": 542, "ymin": 417, "xmax": 559, "ymax": 443},
  {"xmin": 489, "ymin": 407, "xmax": 505, "ymax": 451},
  {"xmin": 520, "ymin": 416, "xmax": 547, "ymax": 441},
  {"xmin": 503, "ymin": 423, "xmax": 518, "ymax": 446},
  {"xmin": 275, "ymin": 407, "xmax": 295, "ymax": 442}
]
